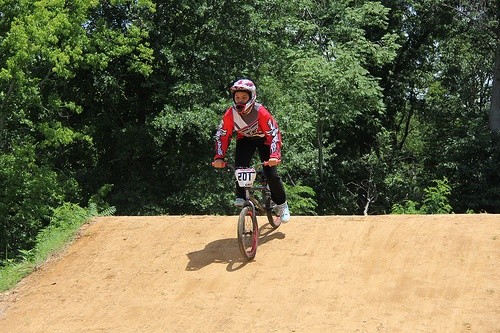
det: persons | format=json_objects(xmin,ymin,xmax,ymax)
[{"xmin": 213, "ymin": 79, "xmax": 291, "ymax": 224}]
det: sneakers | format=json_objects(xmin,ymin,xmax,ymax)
[
  {"xmin": 277, "ymin": 200, "xmax": 290, "ymax": 223},
  {"xmin": 234, "ymin": 198, "xmax": 245, "ymax": 207}
]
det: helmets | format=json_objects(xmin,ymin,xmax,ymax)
[{"xmin": 230, "ymin": 79, "xmax": 257, "ymax": 113}]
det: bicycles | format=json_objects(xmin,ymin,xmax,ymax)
[{"xmin": 211, "ymin": 159, "xmax": 283, "ymax": 260}]
